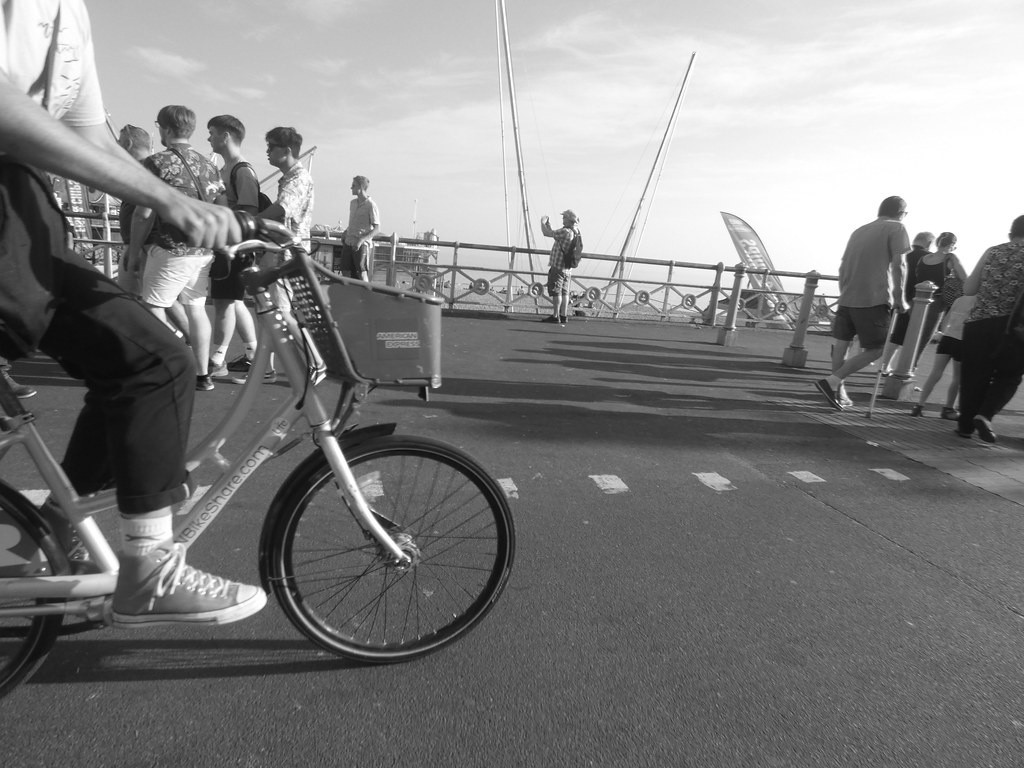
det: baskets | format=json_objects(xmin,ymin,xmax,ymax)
[{"xmin": 244, "ymin": 254, "xmax": 445, "ymax": 388}]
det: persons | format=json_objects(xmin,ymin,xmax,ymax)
[
  {"xmin": 232, "ymin": 127, "xmax": 328, "ymax": 386},
  {"xmin": 126, "ymin": 105, "xmax": 225, "ymax": 391},
  {"xmin": 910, "ymin": 295, "xmax": 976, "ymax": 420},
  {"xmin": 955, "ymin": 216, "xmax": 1024, "ymax": 442},
  {"xmin": 880, "ymin": 230, "xmax": 934, "ymax": 377},
  {"xmin": 342, "ymin": 175, "xmax": 380, "ymax": 282},
  {"xmin": 816, "ymin": 196, "xmax": 910, "ymax": 410},
  {"xmin": 0, "ymin": 0, "xmax": 268, "ymax": 629},
  {"xmin": 208, "ymin": 115, "xmax": 259, "ymax": 376},
  {"xmin": 540, "ymin": 209, "xmax": 579, "ymax": 324},
  {"xmin": 911, "ymin": 232, "xmax": 967, "ymax": 374},
  {"xmin": 119, "ymin": 125, "xmax": 153, "ymax": 301}
]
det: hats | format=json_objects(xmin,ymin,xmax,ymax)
[
  {"xmin": 563, "ymin": 210, "xmax": 580, "ymax": 223},
  {"xmin": 912, "ymin": 232, "xmax": 936, "ymax": 249}
]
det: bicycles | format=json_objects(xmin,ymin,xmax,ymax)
[{"xmin": 0, "ymin": 209, "xmax": 516, "ymax": 698}]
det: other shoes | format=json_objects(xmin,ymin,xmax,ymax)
[
  {"xmin": 815, "ymin": 379, "xmax": 843, "ymax": 410},
  {"xmin": 942, "ymin": 406, "xmax": 960, "ymax": 420},
  {"xmin": 912, "ymin": 404, "xmax": 924, "ymax": 417},
  {"xmin": 954, "ymin": 427, "xmax": 972, "ymax": 438},
  {"xmin": 973, "ymin": 414, "xmax": 997, "ymax": 443}
]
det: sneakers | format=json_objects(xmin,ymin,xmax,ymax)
[
  {"xmin": 32, "ymin": 500, "xmax": 104, "ymax": 580},
  {"xmin": 233, "ymin": 370, "xmax": 278, "ymax": 384},
  {"xmin": 196, "ymin": 376, "xmax": 214, "ymax": 391},
  {"xmin": 207, "ymin": 358, "xmax": 229, "ymax": 376},
  {"xmin": 308, "ymin": 362, "xmax": 327, "ymax": 387},
  {"xmin": 227, "ymin": 358, "xmax": 252, "ymax": 372},
  {"xmin": 543, "ymin": 315, "xmax": 567, "ymax": 323},
  {"xmin": 113, "ymin": 535, "xmax": 267, "ymax": 628},
  {"xmin": 1, "ymin": 370, "xmax": 37, "ymax": 398}
]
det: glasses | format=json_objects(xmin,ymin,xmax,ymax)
[
  {"xmin": 124, "ymin": 124, "xmax": 136, "ymax": 142},
  {"xmin": 154, "ymin": 121, "xmax": 170, "ymax": 129},
  {"xmin": 897, "ymin": 211, "xmax": 908, "ymax": 218},
  {"xmin": 268, "ymin": 142, "xmax": 286, "ymax": 150}
]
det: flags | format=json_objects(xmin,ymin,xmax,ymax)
[{"xmin": 721, "ymin": 211, "xmax": 794, "ymax": 328}]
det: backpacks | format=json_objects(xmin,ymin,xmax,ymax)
[
  {"xmin": 558, "ymin": 226, "xmax": 582, "ymax": 268},
  {"xmin": 230, "ymin": 161, "xmax": 272, "ymax": 215}
]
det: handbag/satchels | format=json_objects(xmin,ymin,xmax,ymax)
[
  {"xmin": 0, "ymin": 154, "xmax": 75, "ymax": 358},
  {"xmin": 212, "ymin": 250, "xmax": 254, "ymax": 300},
  {"xmin": 944, "ymin": 278, "xmax": 964, "ymax": 306}
]
